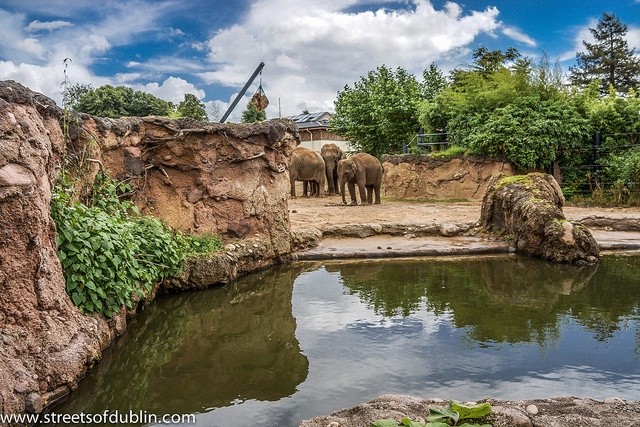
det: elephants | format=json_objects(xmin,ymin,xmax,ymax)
[
  {"xmin": 336, "ymin": 152, "xmax": 382, "ymax": 205},
  {"xmin": 321, "ymin": 142, "xmax": 348, "ymax": 193},
  {"xmin": 288, "ymin": 147, "xmax": 326, "ymax": 197}
]
